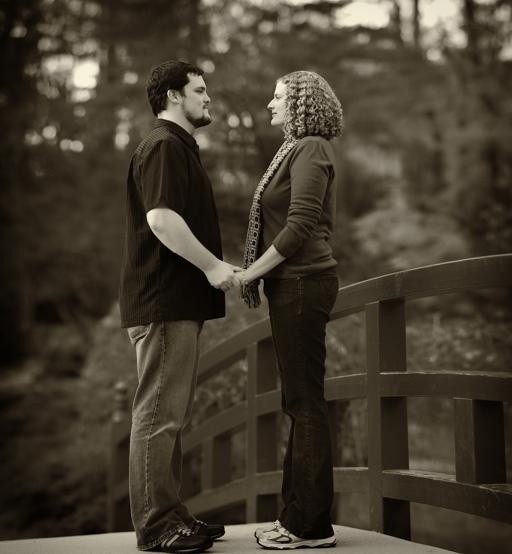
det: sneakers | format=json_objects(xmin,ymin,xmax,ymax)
[
  {"xmin": 252, "ymin": 516, "xmax": 338, "ymax": 548},
  {"xmin": 137, "ymin": 524, "xmax": 217, "ymax": 553},
  {"xmin": 185, "ymin": 517, "xmax": 231, "ymax": 545}
]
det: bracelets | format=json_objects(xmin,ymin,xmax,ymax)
[{"xmin": 240, "ymin": 266, "xmax": 248, "ymax": 285}]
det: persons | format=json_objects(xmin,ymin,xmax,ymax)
[
  {"xmin": 228, "ymin": 70, "xmax": 342, "ymax": 550},
  {"xmin": 121, "ymin": 60, "xmax": 247, "ymax": 552}
]
